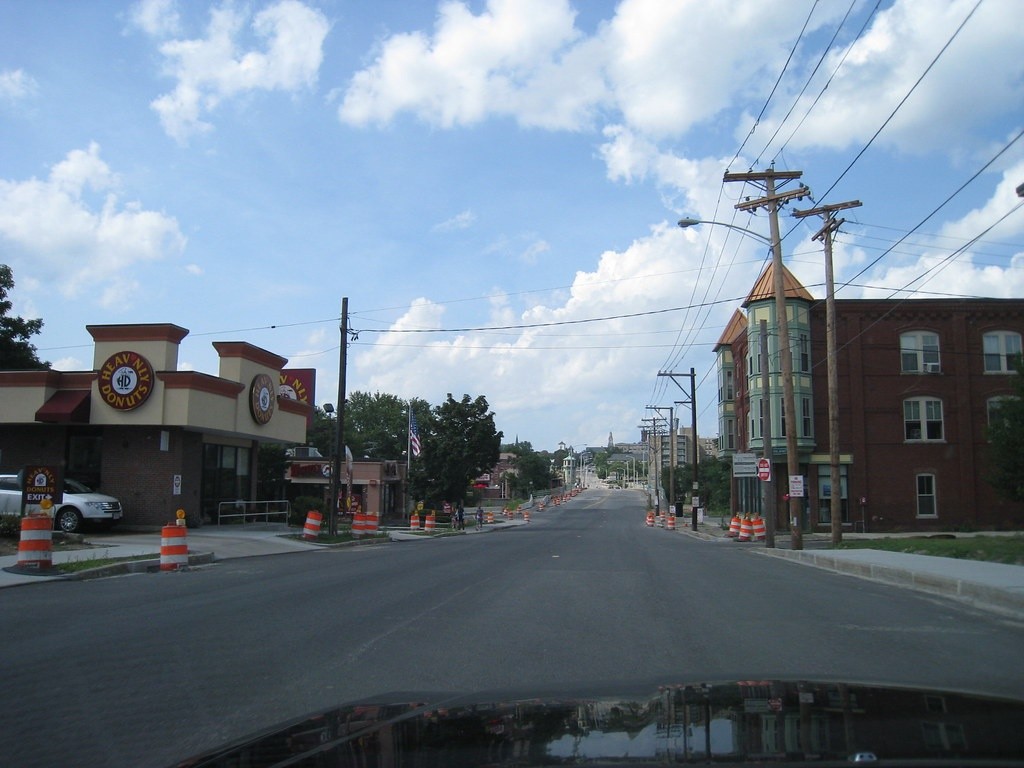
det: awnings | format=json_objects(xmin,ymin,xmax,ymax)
[{"xmin": 35, "ymin": 388, "xmax": 92, "ymax": 424}]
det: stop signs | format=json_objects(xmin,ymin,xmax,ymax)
[{"xmin": 756, "ymin": 458, "xmax": 772, "ymax": 482}]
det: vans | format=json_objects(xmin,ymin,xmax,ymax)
[{"xmin": 0, "ymin": 471, "xmax": 124, "ymax": 534}]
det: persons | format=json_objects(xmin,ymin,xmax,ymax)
[
  {"xmin": 475, "ymin": 504, "xmax": 484, "ymax": 531},
  {"xmin": 457, "ymin": 505, "xmax": 466, "ymax": 530},
  {"xmin": 454, "ymin": 503, "xmax": 463, "ymax": 523}
]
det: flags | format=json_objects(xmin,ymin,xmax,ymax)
[{"xmin": 407, "ymin": 406, "xmax": 424, "ymax": 459}]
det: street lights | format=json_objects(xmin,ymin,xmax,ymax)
[{"xmin": 674, "ymin": 167, "xmax": 806, "ymax": 552}]
